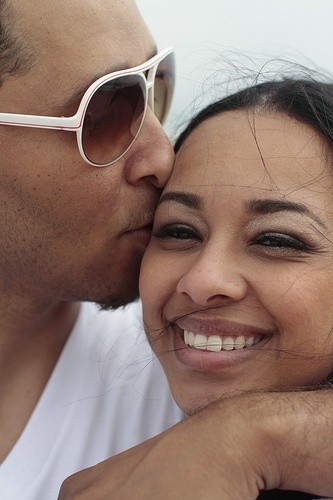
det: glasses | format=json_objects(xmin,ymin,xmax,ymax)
[{"xmin": 0, "ymin": 47, "xmax": 177, "ymax": 168}]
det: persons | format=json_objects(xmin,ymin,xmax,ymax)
[
  {"xmin": 0, "ymin": 0, "xmax": 333, "ymax": 500},
  {"xmin": 138, "ymin": 78, "xmax": 333, "ymax": 414}
]
